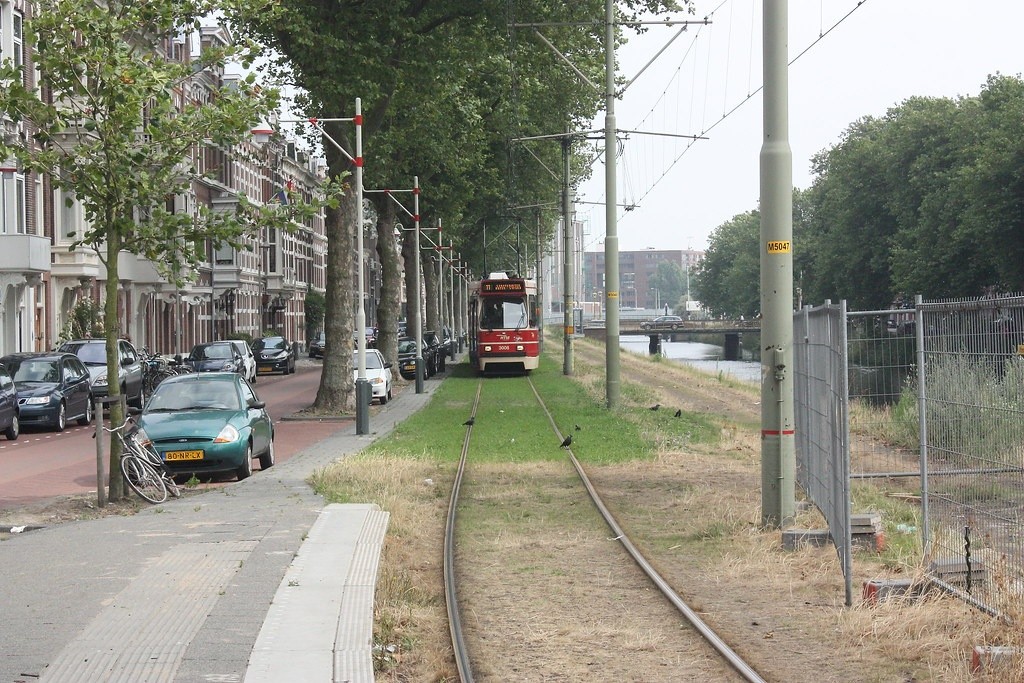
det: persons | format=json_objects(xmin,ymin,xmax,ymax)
[{"xmin": 738, "ymin": 313, "xmax": 744, "ymax": 323}]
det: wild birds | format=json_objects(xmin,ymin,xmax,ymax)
[
  {"xmin": 575, "ymin": 424, "xmax": 581, "ymax": 431},
  {"xmin": 673, "ymin": 409, "xmax": 682, "ymax": 418},
  {"xmin": 559, "ymin": 434, "xmax": 573, "ymax": 450},
  {"xmin": 648, "ymin": 404, "xmax": 660, "ymax": 412},
  {"xmin": 461, "ymin": 416, "xmax": 475, "ymax": 427}
]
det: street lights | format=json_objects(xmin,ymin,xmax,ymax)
[
  {"xmin": 796, "ymin": 287, "xmax": 802, "ymax": 311},
  {"xmin": 593, "ymin": 292, "xmax": 596, "ymax": 320},
  {"xmin": 628, "ymin": 287, "xmax": 638, "ymax": 322},
  {"xmin": 650, "ymin": 287, "xmax": 658, "ymax": 317},
  {"xmin": 598, "ymin": 291, "xmax": 602, "ymax": 320}
]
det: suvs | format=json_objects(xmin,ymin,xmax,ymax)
[
  {"xmin": 640, "ymin": 315, "xmax": 684, "ymax": 330},
  {"xmin": 57, "ymin": 337, "xmax": 144, "ymax": 413}
]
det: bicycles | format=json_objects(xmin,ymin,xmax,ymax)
[
  {"xmin": 138, "ymin": 346, "xmax": 196, "ymax": 398},
  {"xmin": 91, "ymin": 414, "xmax": 180, "ymax": 503}
]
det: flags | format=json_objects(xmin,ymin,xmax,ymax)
[{"xmin": 275, "ymin": 180, "xmax": 294, "ymax": 211}]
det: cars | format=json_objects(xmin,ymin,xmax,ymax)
[
  {"xmin": 897, "ymin": 321, "xmax": 917, "ymax": 336},
  {"xmin": 0, "ymin": 350, "xmax": 94, "ymax": 432},
  {"xmin": 351, "ymin": 348, "xmax": 393, "ymax": 406},
  {"xmin": 353, "ymin": 318, "xmax": 464, "ymax": 380},
  {"xmin": 185, "ymin": 342, "xmax": 247, "ymax": 381},
  {"xmin": 135, "ymin": 371, "xmax": 275, "ymax": 481},
  {"xmin": 887, "ymin": 320, "xmax": 897, "ymax": 328},
  {"xmin": 224, "ymin": 340, "xmax": 257, "ymax": 382},
  {"xmin": 310, "ymin": 331, "xmax": 327, "ymax": 358},
  {"xmin": 0, "ymin": 364, "xmax": 19, "ymax": 441},
  {"xmin": 249, "ymin": 336, "xmax": 296, "ymax": 375}
]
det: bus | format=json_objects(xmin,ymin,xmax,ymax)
[{"xmin": 474, "ymin": 272, "xmax": 541, "ymax": 375}]
